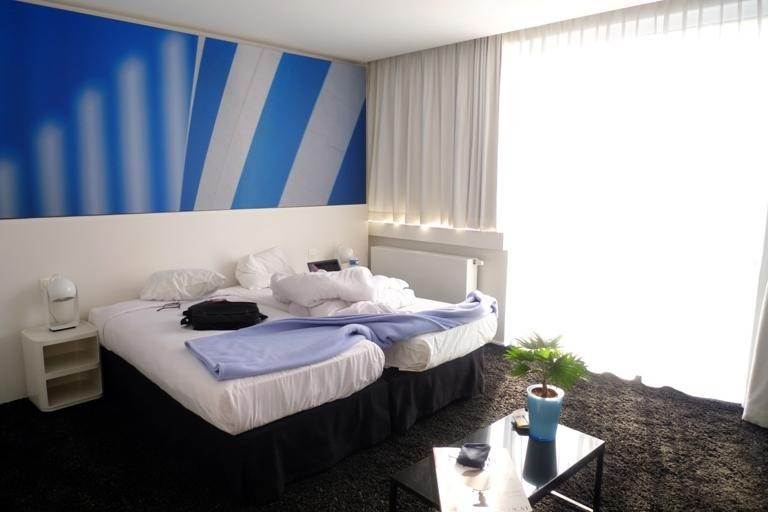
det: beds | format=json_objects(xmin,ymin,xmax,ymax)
[
  {"xmin": 87, "ymin": 267, "xmax": 391, "ymax": 504},
  {"xmin": 225, "ymin": 243, "xmax": 498, "ymax": 427}
]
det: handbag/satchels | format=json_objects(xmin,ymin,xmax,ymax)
[{"xmin": 180, "ymin": 300, "xmax": 267, "ymax": 329}]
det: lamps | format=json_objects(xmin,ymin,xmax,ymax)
[{"xmin": 41, "ymin": 273, "xmax": 81, "ymax": 332}]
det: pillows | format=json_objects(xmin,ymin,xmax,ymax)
[
  {"xmin": 138, "ymin": 267, "xmax": 226, "ymax": 302},
  {"xmin": 235, "ymin": 246, "xmax": 293, "ymax": 290}
]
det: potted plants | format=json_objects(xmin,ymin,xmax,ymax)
[{"xmin": 500, "ymin": 331, "xmax": 590, "ymax": 444}]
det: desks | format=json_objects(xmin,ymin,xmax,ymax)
[{"xmin": 386, "ymin": 407, "xmax": 605, "ymax": 512}]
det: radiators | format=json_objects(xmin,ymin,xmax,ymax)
[{"xmin": 371, "ymin": 245, "xmax": 484, "ymax": 308}]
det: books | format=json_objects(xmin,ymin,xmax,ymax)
[{"xmin": 431, "ymin": 441, "xmax": 532, "ymax": 511}]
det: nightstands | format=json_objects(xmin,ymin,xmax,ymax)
[{"xmin": 20, "ymin": 318, "xmax": 104, "ymax": 414}]
